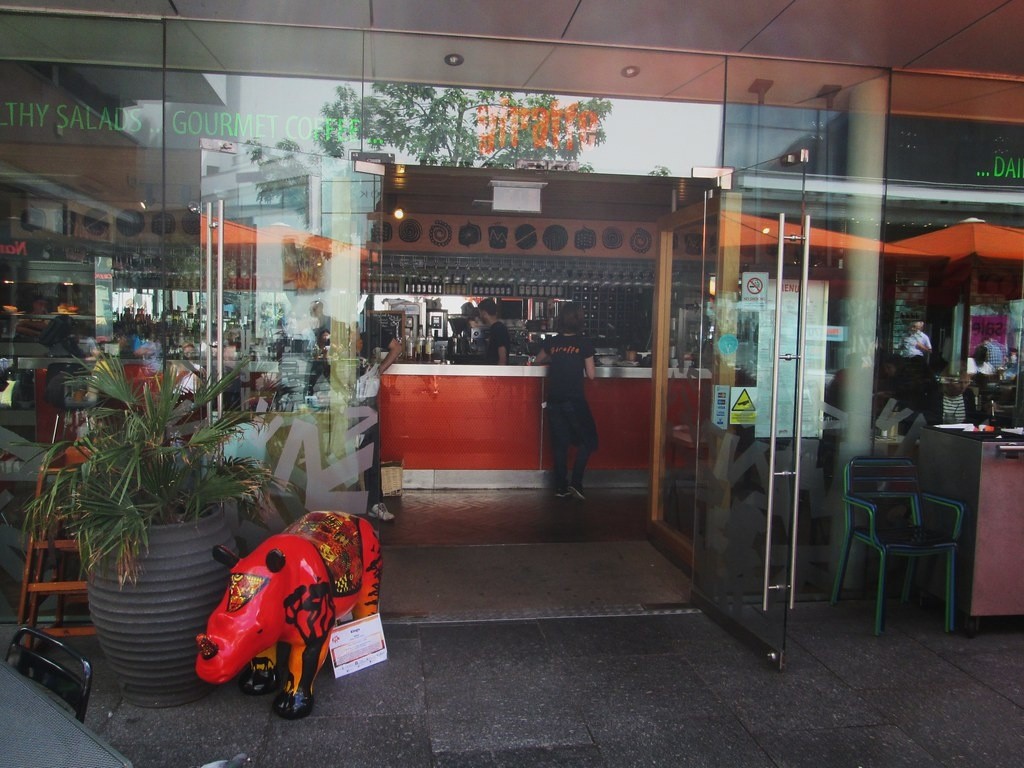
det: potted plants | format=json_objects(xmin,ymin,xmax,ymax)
[{"xmin": 9, "ymin": 338, "xmax": 294, "ymax": 710}]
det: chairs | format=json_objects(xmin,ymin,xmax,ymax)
[
  {"xmin": 5, "ymin": 627, "xmax": 95, "ymax": 725},
  {"xmin": 830, "ymin": 455, "xmax": 965, "ymax": 637}
]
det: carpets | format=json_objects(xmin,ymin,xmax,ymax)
[{"xmin": 375, "ymin": 540, "xmax": 689, "ymax": 617}]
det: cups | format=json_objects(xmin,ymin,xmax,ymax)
[
  {"xmin": 440, "ymin": 346, "xmax": 446, "ymax": 361},
  {"xmin": 625, "ymin": 351, "xmax": 636, "ymax": 362}
]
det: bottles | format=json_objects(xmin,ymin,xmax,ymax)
[
  {"xmin": 425, "ymin": 327, "xmax": 434, "ymax": 361},
  {"xmin": 406, "ymin": 327, "xmax": 414, "ymax": 361},
  {"xmin": 113, "ymin": 305, "xmax": 200, "ymax": 349},
  {"xmin": 371, "ymin": 267, "xmax": 562, "ymax": 299},
  {"xmin": 415, "ymin": 325, "xmax": 425, "ymax": 361}
]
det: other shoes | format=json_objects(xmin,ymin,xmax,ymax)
[
  {"xmin": 553, "ymin": 486, "xmax": 574, "ymax": 498},
  {"xmin": 568, "ymin": 480, "xmax": 586, "ymax": 500}
]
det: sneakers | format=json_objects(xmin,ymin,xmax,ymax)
[{"xmin": 368, "ymin": 502, "xmax": 396, "ymax": 521}]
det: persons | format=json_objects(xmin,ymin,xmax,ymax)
[
  {"xmin": 898, "ymin": 321, "xmax": 931, "ymax": 359},
  {"xmin": 478, "ymin": 298, "xmax": 509, "ymax": 365},
  {"xmin": 534, "ymin": 303, "xmax": 595, "ymax": 502},
  {"xmin": 359, "ymin": 313, "xmax": 403, "ymax": 522},
  {"xmin": 302, "ymin": 301, "xmax": 351, "ymax": 403},
  {"xmin": 46, "ymin": 303, "xmax": 258, "ymax": 415},
  {"xmin": 835, "ymin": 336, "xmax": 1021, "ymax": 424}
]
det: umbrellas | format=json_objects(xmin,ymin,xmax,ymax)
[
  {"xmin": 198, "ymin": 215, "xmax": 380, "ymax": 295},
  {"xmin": 719, "ymin": 208, "xmax": 947, "ymax": 275},
  {"xmin": 889, "ymin": 222, "xmax": 1023, "ymax": 284}
]
